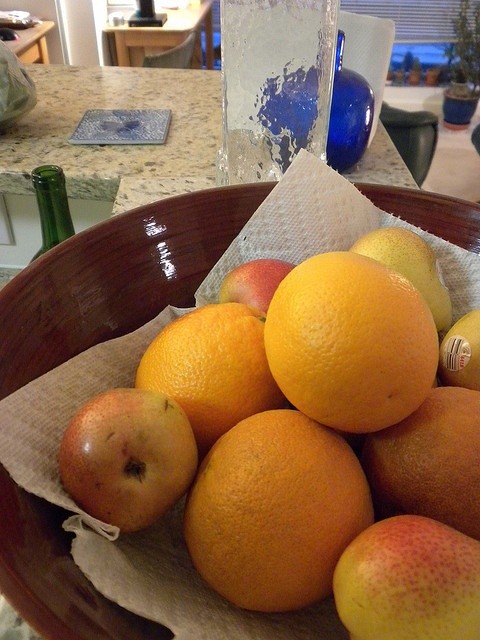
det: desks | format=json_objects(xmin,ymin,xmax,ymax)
[
  {"xmin": 1, "ymin": 21, "xmax": 55, "ymax": 62},
  {"xmin": 103, "ymin": 2, "xmax": 215, "ymax": 70}
]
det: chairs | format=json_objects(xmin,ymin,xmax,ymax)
[
  {"xmin": 378, "ymin": 101, "xmax": 440, "ymax": 189},
  {"xmin": 144, "ymin": 32, "xmax": 195, "ymax": 67}
]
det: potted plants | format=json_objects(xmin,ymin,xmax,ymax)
[
  {"xmin": 442, "ymin": 1, "xmax": 480, "ymax": 131},
  {"xmin": 386, "ymin": 57, "xmax": 443, "ymax": 87},
  {"xmin": 448, "ymin": 63, "xmax": 469, "ymax": 87}
]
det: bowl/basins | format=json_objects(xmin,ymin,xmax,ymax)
[{"xmin": 1, "ymin": 179, "xmax": 479, "ymax": 640}]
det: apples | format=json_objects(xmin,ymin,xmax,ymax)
[
  {"xmin": 219, "ymin": 258, "xmax": 298, "ymax": 316},
  {"xmin": 439, "ymin": 310, "xmax": 480, "ymax": 394},
  {"xmin": 332, "ymin": 513, "xmax": 479, "ymax": 636},
  {"xmin": 61, "ymin": 386, "xmax": 199, "ymax": 534},
  {"xmin": 348, "ymin": 227, "xmax": 451, "ymax": 331}
]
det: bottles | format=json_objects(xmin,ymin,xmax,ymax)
[{"xmin": 28, "ymin": 164, "xmax": 78, "ymax": 265}]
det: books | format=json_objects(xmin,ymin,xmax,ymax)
[{"xmin": 68, "ymin": 108, "xmax": 172, "ymax": 145}]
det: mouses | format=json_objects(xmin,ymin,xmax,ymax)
[{"xmin": 0, "ymin": 28, "xmax": 19, "ymax": 41}]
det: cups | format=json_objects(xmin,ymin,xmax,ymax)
[{"xmin": 220, "ymin": 0, "xmax": 340, "ymax": 187}]
